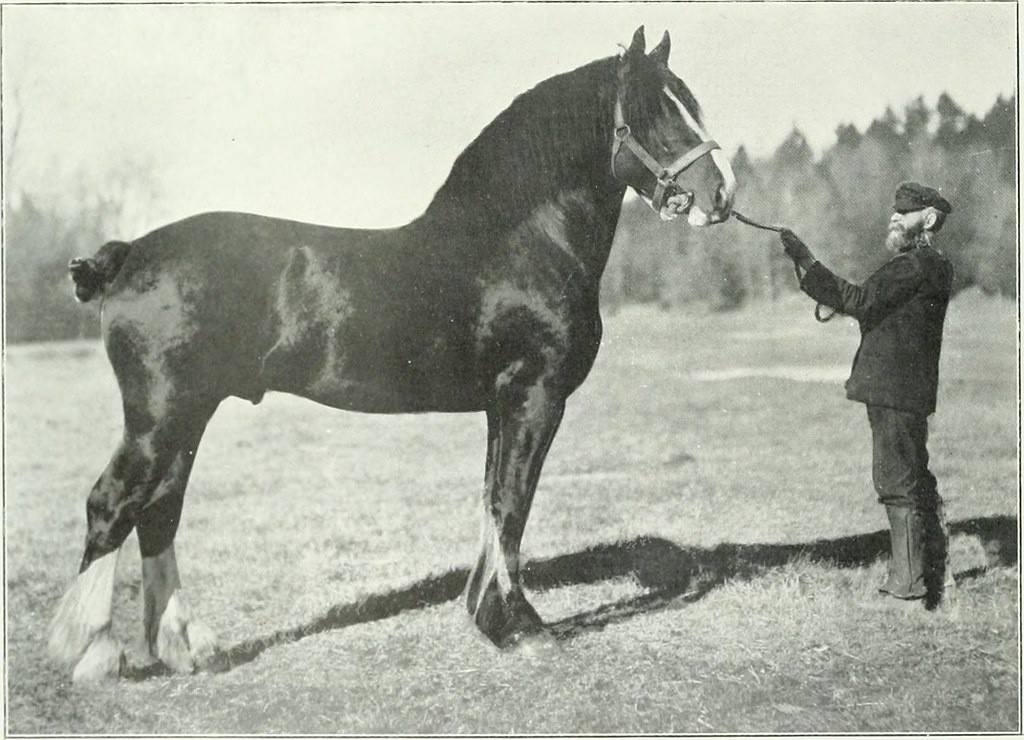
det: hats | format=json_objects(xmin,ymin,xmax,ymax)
[{"xmin": 893, "ymin": 182, "xmax": 952, "ymax": 215}]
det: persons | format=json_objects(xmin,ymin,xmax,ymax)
[{"xmin": 780, "ymin": 182, "xmax": 954, "ymax": 601}]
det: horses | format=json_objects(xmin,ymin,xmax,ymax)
[{"xmin": 37, "ymin": 26, "xmax": 740, "ymax": 695}]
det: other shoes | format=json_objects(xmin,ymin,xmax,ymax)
[
  {"xmin": 856, "ymin": 590, "xmax": 928, "ymax": 613},
  {"xmin": 926, "ymin": 571, "xmax": 959, "ymax": 604}
]
